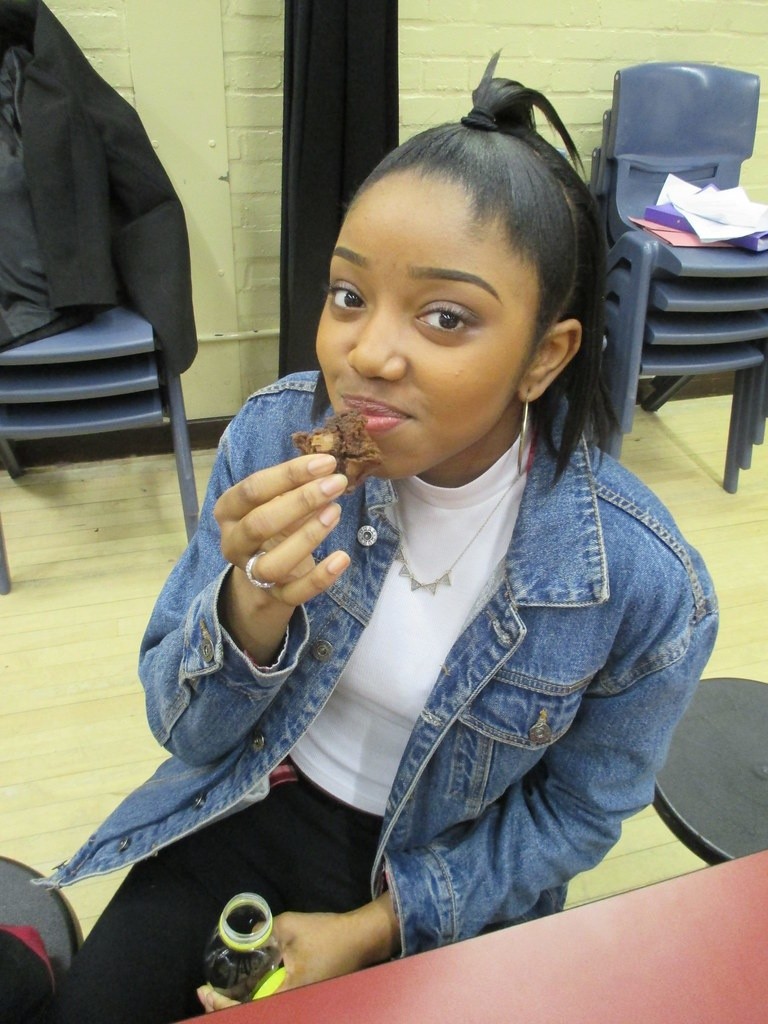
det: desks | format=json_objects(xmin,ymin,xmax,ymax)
[{"xmin": 178, "ymin": 852, "xmax": 768, "ymax": 1024}]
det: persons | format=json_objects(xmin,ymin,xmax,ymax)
[{"xmin": 30, "ymin": 79, "xmax": 720, "ymax": 1023}]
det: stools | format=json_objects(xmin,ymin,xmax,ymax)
[
  {"xmin": 1, "ymin": 858, "xmax": 82, "ymax": 989},
  {"xmin": 657, "ymin": 678, "xmax": 766, "ymax": 859}
]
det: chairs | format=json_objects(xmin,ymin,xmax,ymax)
[
  {"xmin": 0, "ymin": 303, "xmax": 202, "ymax": 594},
  {"xmin": 586, "ymin": 61, "xmax": 768, "ymax": 494}
]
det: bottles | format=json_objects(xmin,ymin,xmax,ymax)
[{"xmin": 203, "ymin": 890, "xmax": 288, "ymax": 1005}]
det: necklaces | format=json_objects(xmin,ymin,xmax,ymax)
[{"xmin": 389, "ymin": 472, "xmax": 523, "ymax": 594}]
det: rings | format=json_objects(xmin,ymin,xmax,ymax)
[{"xmin": 245, "ymin": 551, "xmax": 275, "ymax": 589}]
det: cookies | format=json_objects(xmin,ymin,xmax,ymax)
[{"xmin": 292, "ymin": 406, "xmax": 383, "ymax": 497}]
